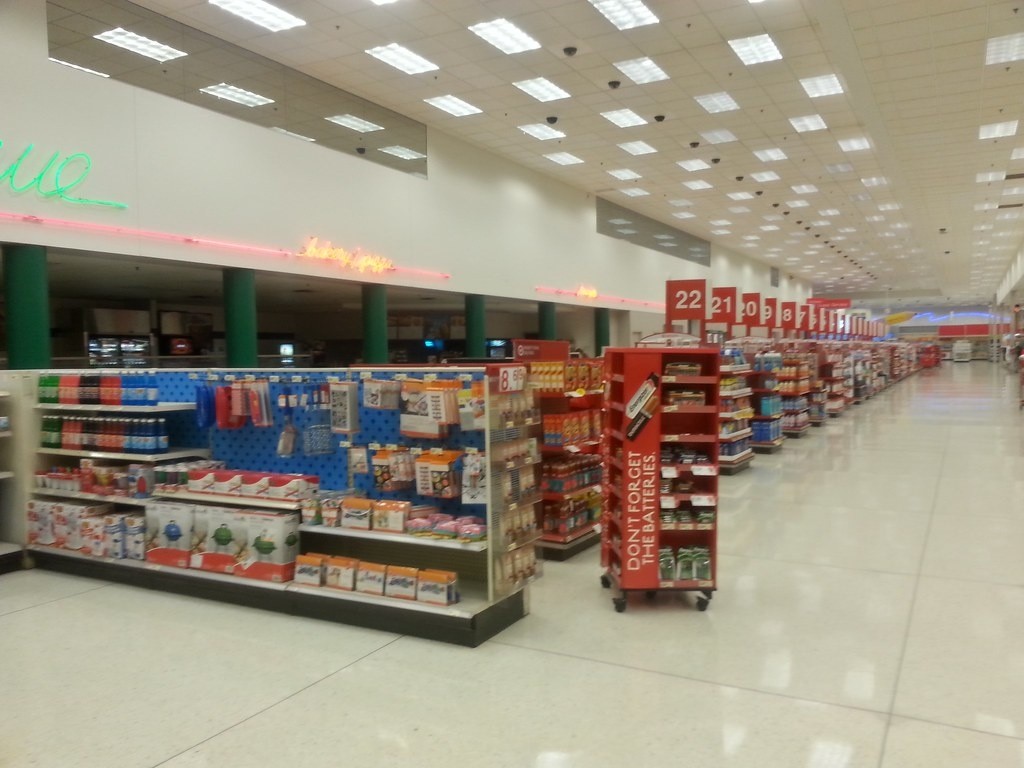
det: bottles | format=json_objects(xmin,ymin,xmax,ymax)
[
  {"xmin": 718, "ymin": 349, "xmax": 808, "ymax": 460},
  {"xmin": 40, "ymin": 414, "xmax": 170, "ymax": 455},
  {"xmin": 38, "ymin": 371, "xmax": 160, "ymax": 407},
  {"xmin": 540, "ymin": 452, "xmax": 603, "ymax": 534}
]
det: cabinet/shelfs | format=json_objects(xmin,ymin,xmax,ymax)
[
  {"xmin": 534, "ymin": 330, "xmax": 946, "ymax": 614},
  {"xmin": 0, "ymin": 357, "xmax": 548, "ymax": 649}
]
